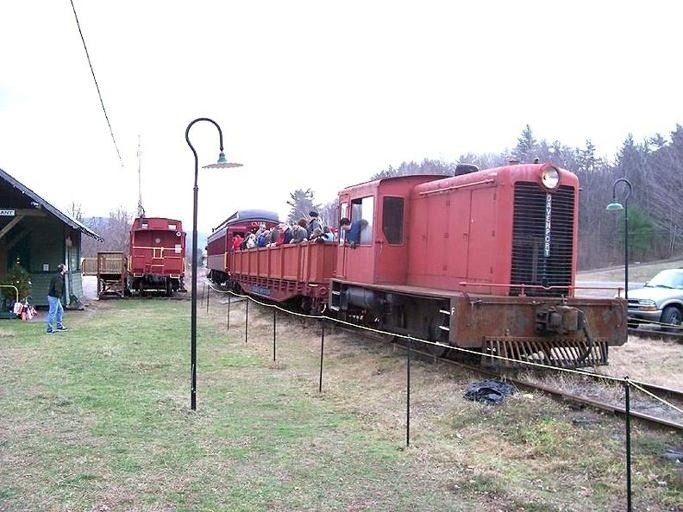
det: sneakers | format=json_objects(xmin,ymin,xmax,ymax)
[{"xmin": 47, "ymin": 326, "xmax": 68, "ymax": 334}]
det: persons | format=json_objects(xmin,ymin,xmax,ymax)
[
  {"xmin": 337, "ymin": 217, "xmax": 361, "ymax": 248},
  {"xmin": 229, "ymin": 210, "xmax": 339, "ymax": 253},
  {"xmin": 45, "ymin": 263, "xmax": 68, "ymax": 334}
]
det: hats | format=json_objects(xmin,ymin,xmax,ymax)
[{"xmin": 309, "ymin": 210, "xmax": 319, "ymax": 216}]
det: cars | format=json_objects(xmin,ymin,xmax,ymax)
[{"xmin": 618, "ymin": 268, "xmax": 683, "ymax": 338}]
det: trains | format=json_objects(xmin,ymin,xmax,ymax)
[
  {"xmin": 129, "ymin": 216, "xmax": 187, "ymax": 298},
  {"xmin": 207, "ymin": 162, "xmax": 628, "ymax": 365}
]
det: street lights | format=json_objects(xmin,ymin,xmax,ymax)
[
  {"xmin": 607, "ymin": 177, "xmax": 632, "ymax": 345},
  {"xmin": 185, "ymin": 118, "xmax": 242, "ymax": 409}
]
